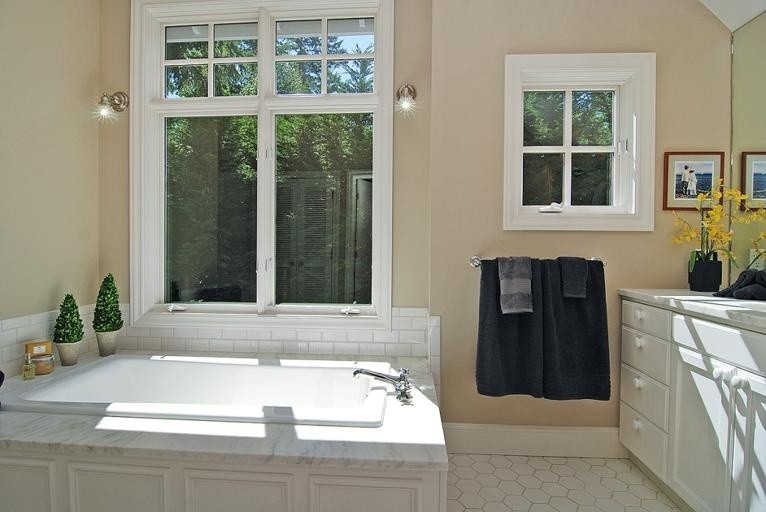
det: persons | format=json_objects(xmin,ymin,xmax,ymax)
[
  {"xmin": 681, "ymin": 165, "xmax": 689, "ymax": 194},
  {"xmin": 688, "ymin": 169, "xmax": 698, "ymax": 195}
]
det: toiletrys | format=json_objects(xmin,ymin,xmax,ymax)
[{"xmin": 20, "ymin": 351, "xmax": 35, "ymax": 380}]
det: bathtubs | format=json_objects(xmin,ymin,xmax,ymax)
[{"xmin": 0, "ymin": 354, "xmax": 392, "ymax": 427}]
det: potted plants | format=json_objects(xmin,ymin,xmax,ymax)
[
  {"xmin": 92, "ymin": 273, "xmax": 125, "ymax": 356},
  {"xmin": 53, "ymin": 294, "xmax": 85, "ymax": 366}
]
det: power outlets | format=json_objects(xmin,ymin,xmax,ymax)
[{"xmin": 750, "ymin": 249, "xmax": 764, "ymax": 271}]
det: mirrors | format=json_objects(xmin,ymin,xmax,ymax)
[{"xmin": 728, "ymin": 10, "xmax": 766, "ymax": 289}]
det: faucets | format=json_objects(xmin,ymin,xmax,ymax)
[{"xmin": 348, "ymin": 368, "xmax": 410, "ymax": 394}]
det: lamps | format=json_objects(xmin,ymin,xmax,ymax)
[
  {"xmin": 397, "ymin": 83, "xmax": 417, "ymax": 109},
  {"xmin": 97, "ymin": 92, "xmax": 128, "ymax": 116}
]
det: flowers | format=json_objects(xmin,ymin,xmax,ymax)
[
  {"xmin": 731, "ymin": 195, "xmax": 766, "ymax": 270},
  {"xmin": 669, "ymin": 176, "xmax": 763, "ymax": 273}
]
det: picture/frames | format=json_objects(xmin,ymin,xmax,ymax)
[
  {"xmin": 663, "ymin": 152, "xmax": 724, "ymax": 211},
  {"xmin": 740, "ymin": 151, "xmax": 766, "ymax": 212}
]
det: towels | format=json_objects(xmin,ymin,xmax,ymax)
[
  {"xmin": 557, "ymin": 256, "xmax": 588, "ymax": 298},
  {"xmin": 712, "ymin": 269, "xmax": 758, "ymax": 297},
  {"xmin": 734, "ymin": 284, "xmax": 766, "ymax": 301},
  {"xmin": 476, "ymin": 258, "xmax": 543, "ymax": 398},
  {"xmin": 541, "ymin": 259, "xmax": 611, "ymax": 401},
  {"xmin": 495, "ymin": 257, "xmax": 534, "ymax": 315},
  {"xmin": 755, "ymin": 270, "xmax": 766, "ymax": 286}
]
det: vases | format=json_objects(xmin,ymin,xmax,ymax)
[{"xmin": 688, "ymin": 260, "xmax": 722, "ymax": 292}]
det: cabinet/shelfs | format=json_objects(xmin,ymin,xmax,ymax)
[
  {"xmin": 276, "ymin": 171, "xmax": 346, "ymax": 303},
  {"xmin": 616, "ymin": 288, "xmax": 766, "ymax": 512}
]
